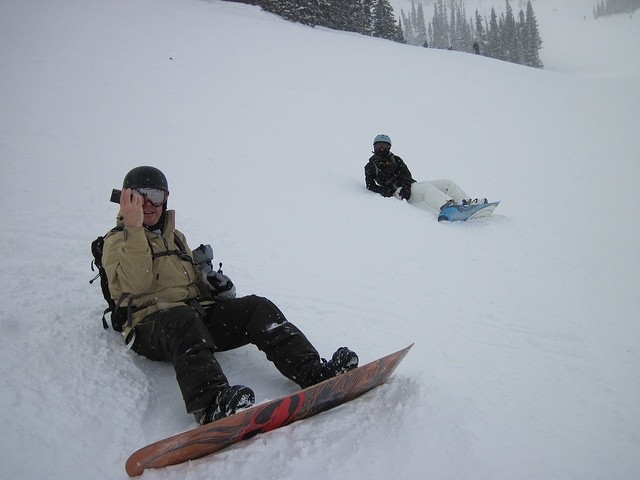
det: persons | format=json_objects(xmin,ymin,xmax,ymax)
[
  {"xmin": 364, "ymin": 134, "xmax": 488, "ymax": 212},
  {"xmin": 101, "ymin": 166, "xmax": 359, "ymax": 425}
]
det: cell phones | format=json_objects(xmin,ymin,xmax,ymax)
[{"xmin": 109, "ymin": 188, "xmax": 133, "ymax": 205}]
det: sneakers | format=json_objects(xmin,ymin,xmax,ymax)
[
  {"xmin": 200, "ymin": 385, "xmax": 254, "ymax": 426},
  {"xmin": 472, "ymin": 198, "xmax": 488, "ymax": 204},
  {"xmin": 302, "ymin": 348, "xmax": 359, "ymax": 383}
]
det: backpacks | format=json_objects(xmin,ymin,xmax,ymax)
[{"xmin": 89, "ymin": 227, "xmax": 206, "ymax": 331}]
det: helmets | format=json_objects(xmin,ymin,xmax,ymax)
[
  {"xmin": 374, "ymin": 135, "xmax": 391, "ymax": 145},
  {"xmin": 123, "ymin": 166, "xmax": 168, "ymax": 192}
]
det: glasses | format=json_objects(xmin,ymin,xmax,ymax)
[{"xmin": 130, "ymin": 187, "xmax": 166, "ymax": 206}]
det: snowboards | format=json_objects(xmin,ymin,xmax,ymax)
[
  {"xmin": 125, "ymin": 342, "xmax": 416, "ymax": 478},
  {"xmin": 437, "ymin": 201, "xmax": 500, "ymax": 222}
]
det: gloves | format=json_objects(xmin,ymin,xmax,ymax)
[
  {"xmin": 399, "ymin": 180, "xmax": 411, "ymax": 200},
  {"xmin": 380, "ymin": 186, "xmax": 397, "ymax": 198}
]
